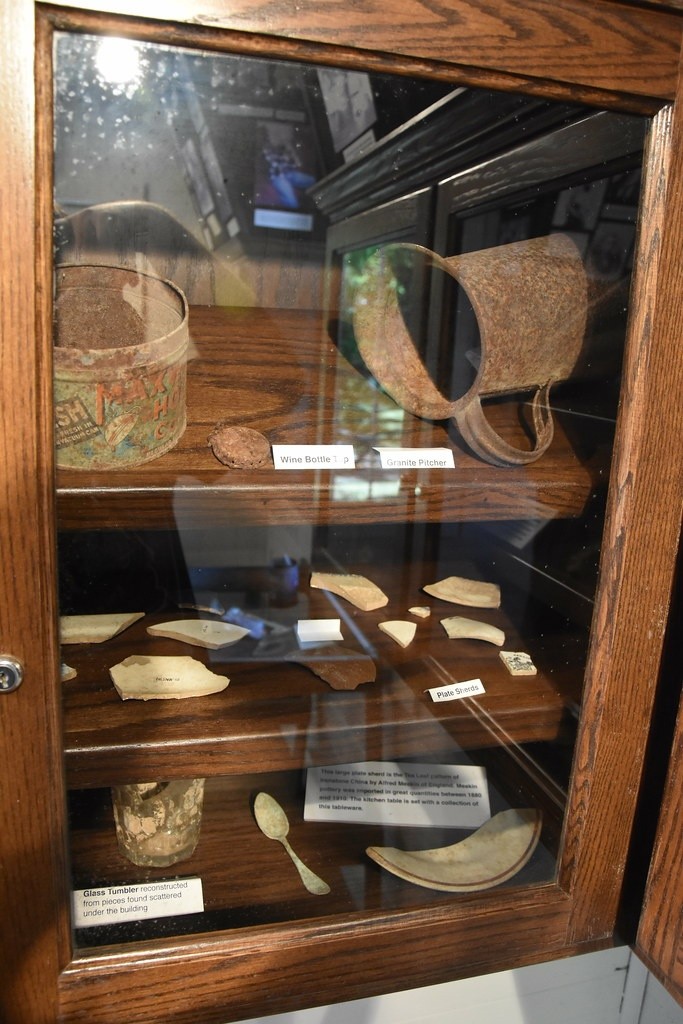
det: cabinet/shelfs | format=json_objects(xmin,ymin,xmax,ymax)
[{"xmin": 0, "ymin": 0, "xmax": 683, "ymax": 1024}]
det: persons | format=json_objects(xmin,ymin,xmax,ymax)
[{"xmin": 54, "ymin": 199, "xmax": 303, "ymax": 678}]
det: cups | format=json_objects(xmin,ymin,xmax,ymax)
[
  {"xmin": 353, "ymin": 232, "xmax": 629, "ymax": 468},
  {"xmin": 112, "ymin": 778, "xmax": 205, "ymax": 868}
]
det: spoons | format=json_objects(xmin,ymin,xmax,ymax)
[{"xmin": 254, "ymin": 792, "xmax": 331, "ymax": 895}]
